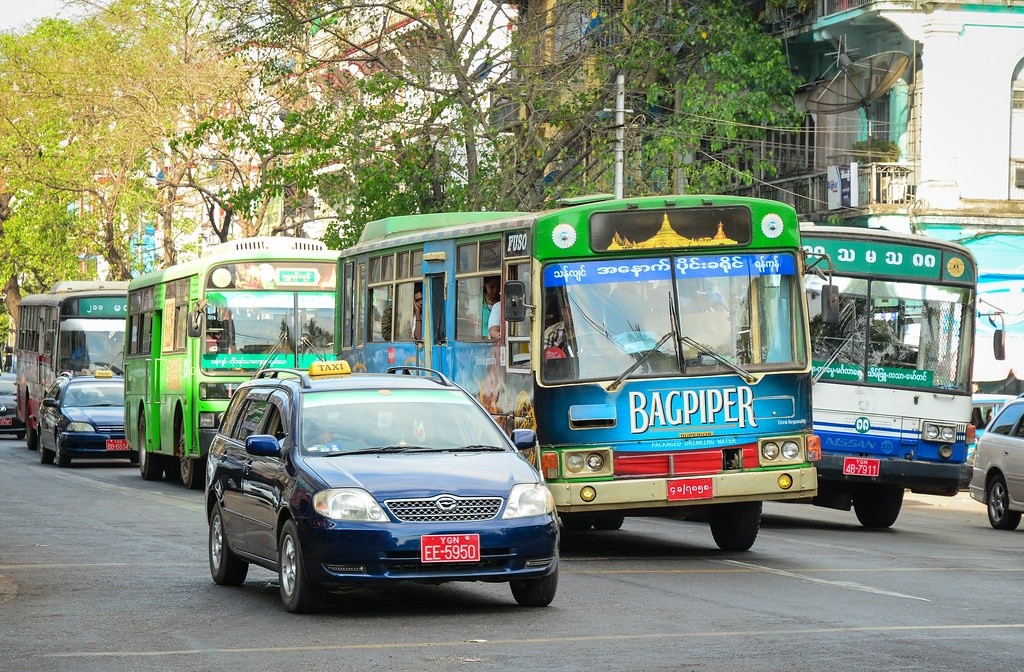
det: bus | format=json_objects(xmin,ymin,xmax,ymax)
[
  {"xmin": 14, "ymin": 280, "xmax": 129, "ymax": 451},
  {"xmin": 329, "ymin": 190, "xmax": 840, "ymax": 551},
  {"xmin": 122, "ymin": 235, "xmax": 342, "ymax": 489},
  {"xmin": 756, "ymin": 219, "xmax": 1008, "ymax": 529}
]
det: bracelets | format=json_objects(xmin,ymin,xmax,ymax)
[{"xmin": 415, "ymin": 320, "xmax": 421, "ymax": 321}]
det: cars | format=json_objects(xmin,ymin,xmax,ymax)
[
  {"xmin": 35, "ymin": 368, "xmax": 140, "ymax": 467},
  {"xmin": 969, "ymin": 394, "xmax": 1024, "ymax": 530},
  {"xmin": 957, "ymin": 391, "xmax": 1018, "ymax": 489},
  {"xmin": 0, "ymin": 371, "xmax": 27, "ymax": 439},
  {"xmin": 205, "ymin": 361, "xmax": 563, "ymax": 615}
]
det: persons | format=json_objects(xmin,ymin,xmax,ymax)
[
  {"xmin": 305, "ymin": 407, "xmax": 365, "ymax": 447},
  {"xmin": 411, "ymin": 290, "xmax": 423, "ymax": 340},
  {"xmin": 381, "ymin": 304, "xmax": 392, "ymax": 341},
  {"xmin": 71, "ymin": 334, "xmax": 100, "ymax": 361},
  {"xmin": 544, "ymin": 296, "xmax": 590, "ymax": 348},
  {"xmin": 469, "ymin": 277, "xmax": 501, "ymax": 339},
  {"xmin": 970, "ymin": 407, "xmax": 992, "ymax": 429}
]
